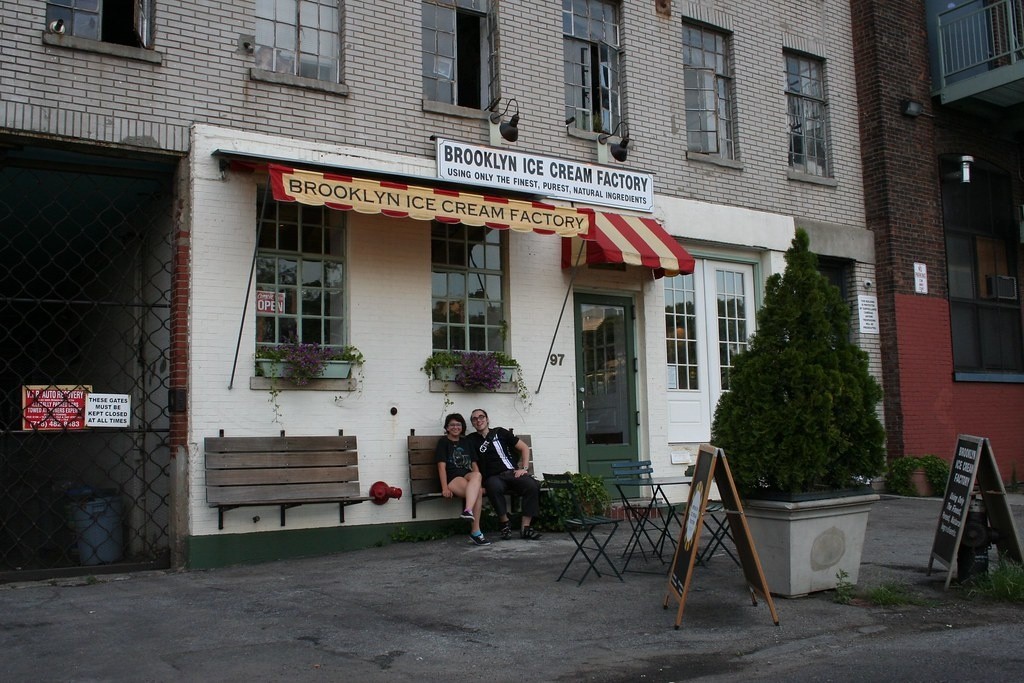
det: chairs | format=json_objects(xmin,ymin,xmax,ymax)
[
  {"xmin": 541, "ymin": 472, "xmax": 624, "ymax": 588},
  {"xmin": 611, "ymin": 459, "xmax": 683, "ymax": 564},
  {"xmin": 684, "ymin": 464, "xmax": 742, "ymax": 570}
]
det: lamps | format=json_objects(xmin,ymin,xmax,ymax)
[
  {"xmin": 598, "ymin": 121, "xmax": 630, "ymax": 162},
  {"xmin": 490, "ymin": 99, "xmax": 520, "ymax": 143},
  {"xmin": 901, "ymin": 99, "xmax": 923, "ymax": 116}
]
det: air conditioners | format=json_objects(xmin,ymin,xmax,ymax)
[{"xmin": 986, "ymin": 274, "xmax": 1017, "ymax": 301}]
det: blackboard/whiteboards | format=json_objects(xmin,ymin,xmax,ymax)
[
  {"xmin": 669, "ymin": 444, "xmax": 770, "ymax": 604},
  {"xmin": 929, "ymin": 433, "xmax": 1024, "ymax": 570}
]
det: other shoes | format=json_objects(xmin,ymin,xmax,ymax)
[
  {"xmin": 500, "ymin": 512, "xmax": 512, "ymax": 540},
  {"xmin": 520, "ymin": 526, "xmax": 543, "ymax": 540}
]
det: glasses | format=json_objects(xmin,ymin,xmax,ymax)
[
  {"xmin": 471, "ymin": 415, "xmax": 486, "ymax": 422},
  {"xmin": 447, "ymin": 423, "xmax": 463, "ymax": 428}
]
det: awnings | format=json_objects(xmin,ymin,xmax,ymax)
[
  {"xmin": 221, "ymin": 157, "xmax": 596, "ymax": 245},
  {"xmin": 560, "ymin": 208, "xmax": 697, "ymax": 281}
]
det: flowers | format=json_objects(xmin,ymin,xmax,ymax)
[
  {"xmin": 421, "ymin": 348, "xmax": 535, "ymax": 409},
  {"xmin": 250, "ymin": 334, "xmax": 367, "ymax": 422}
]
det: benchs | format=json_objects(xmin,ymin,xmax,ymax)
[
  {"xmin": 408, "ymin": 428, "xmax": 539, "ymax": 518},
  {"xmin": 203, "ymin": 428, "xmax": 375, "ymax": 530}
]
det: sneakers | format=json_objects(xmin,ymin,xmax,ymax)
[
  {"xmin": 468, "ymin": 532, "xmax": 490, "ymax": 546},
  {"xmin": 460, "ymin": 511, "xmax": 475, "ymax": 522}
]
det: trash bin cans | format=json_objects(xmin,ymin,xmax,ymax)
[{"xmin": 70, "ymin": 487, "xmax": 125, "ymax": 564}]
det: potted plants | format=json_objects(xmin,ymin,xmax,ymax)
[
  {"xmin": 720, "ymin": 224, "xmax": 888, "ymax": 597},
  {"xmin": 891, "ymin": 452, "xmax": 952, "ymax": 498}
]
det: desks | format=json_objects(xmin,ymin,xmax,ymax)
[{"xmin": 603, "ymin": 476, "xmax": 706, "ymax": 566}]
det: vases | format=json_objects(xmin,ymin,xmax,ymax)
[
  {"xmin": 255, "ymin": 357, "xmax": 351, "ymax": 379},
  {"xmin": 429, "ymin": 362, "xmax": 519, "ymax": 393}
]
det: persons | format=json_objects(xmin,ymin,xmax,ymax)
[
  {"xmin": 435, "ymin": 414, "xmax": 491, "ymax": 548},
  {"xmin": 467, "ymin": 408, "xmax": 544, "ymax": 540}
]
